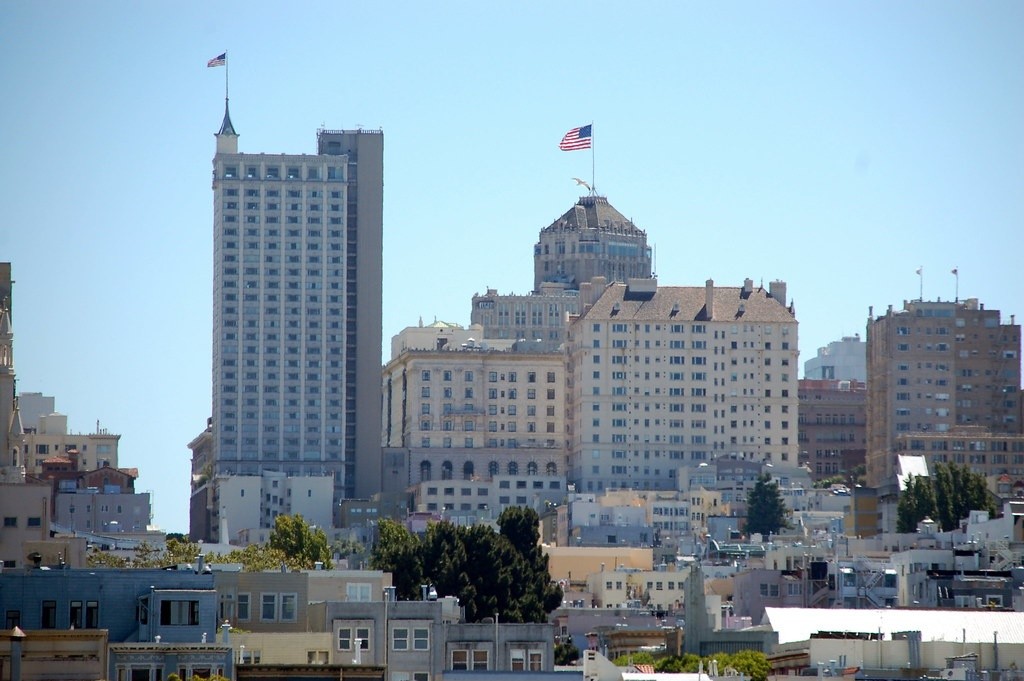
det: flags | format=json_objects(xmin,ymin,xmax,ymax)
[
  {"xmin": 559, "ymin": 124, "xmax": 591, "ymax": 151},
  {"xmin": 208, "ymin": 52, "xmax": 225, "ymax": 67}
]
